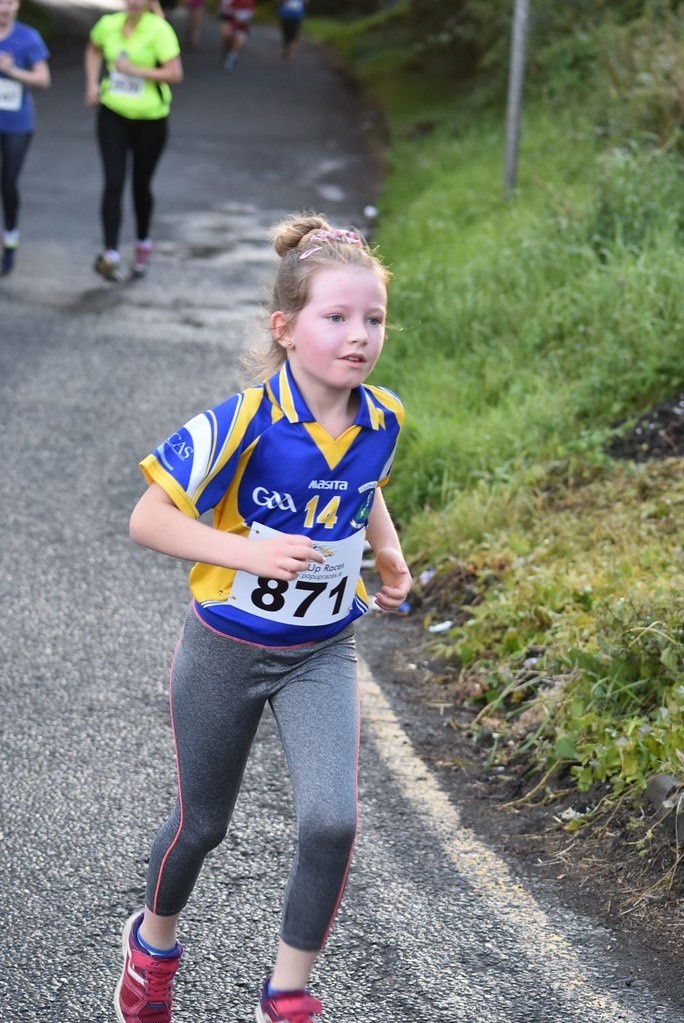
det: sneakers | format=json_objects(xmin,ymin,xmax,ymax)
[
  {"xmin": 113, "ymin": 910, "xmax": 183, "ymax": 1022},
  {"xmin": 254, "ymin": 975, "xmax": 322, "ymax": 1023},
  {"xmin": 131, "ymin": 236, "xmax": 153, "ymax": 277},
  {"xmin": 0, "ymin": 244, "xmax": 17, "ymax": 275},
  {"xmin": 92, "ymin": 248, "xmax": 123, "ymax": 283}
]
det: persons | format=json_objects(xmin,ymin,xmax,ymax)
[
  {"xmin": 1, "ymin": 0, "xmax": 302, "ymax": 278},
  {"xmin": 117, "ymin": 217, "xmax": 415, "ymax": 1022}
]
